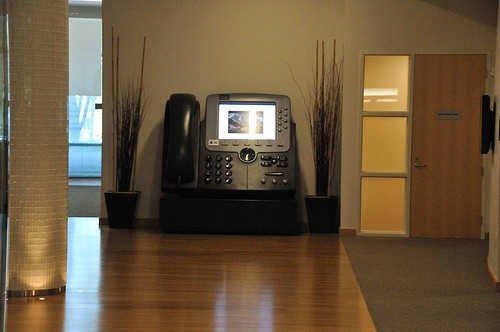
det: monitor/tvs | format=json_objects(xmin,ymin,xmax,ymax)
[{"xmin": 206, "ymin": 93, "xmax": 292, "ymax": 163}]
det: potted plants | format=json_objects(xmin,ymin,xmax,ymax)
[
  {"xmin": 103, "ymin": 25, "xmax": 151, "ymax": 230},
  {"xmin": 281, "ymin": 39, "xmax": 345, "ymax": 235}
]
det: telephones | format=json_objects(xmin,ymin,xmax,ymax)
[{"xmin": 160, "ymin": 89, "xmax": 297, "ymax": 197}]
